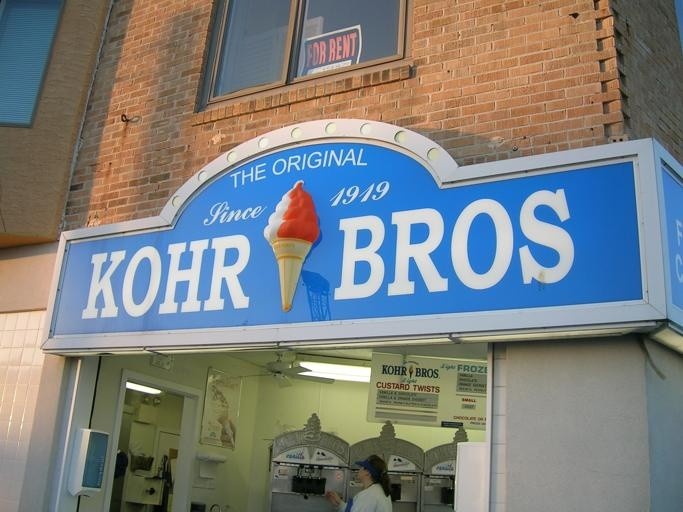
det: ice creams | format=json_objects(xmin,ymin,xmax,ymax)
[{"xmin": 263, "ymin": 180, "xmax": 319, "ymax": 312}]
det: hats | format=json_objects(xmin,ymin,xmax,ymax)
[{"xmin": 355, "ymin": 459, "xmax": 380, "ymax": 480}]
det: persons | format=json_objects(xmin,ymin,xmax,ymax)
[{"xmin": 326, "ymin": 455, "xmax": 392, "ymax": 512}]
